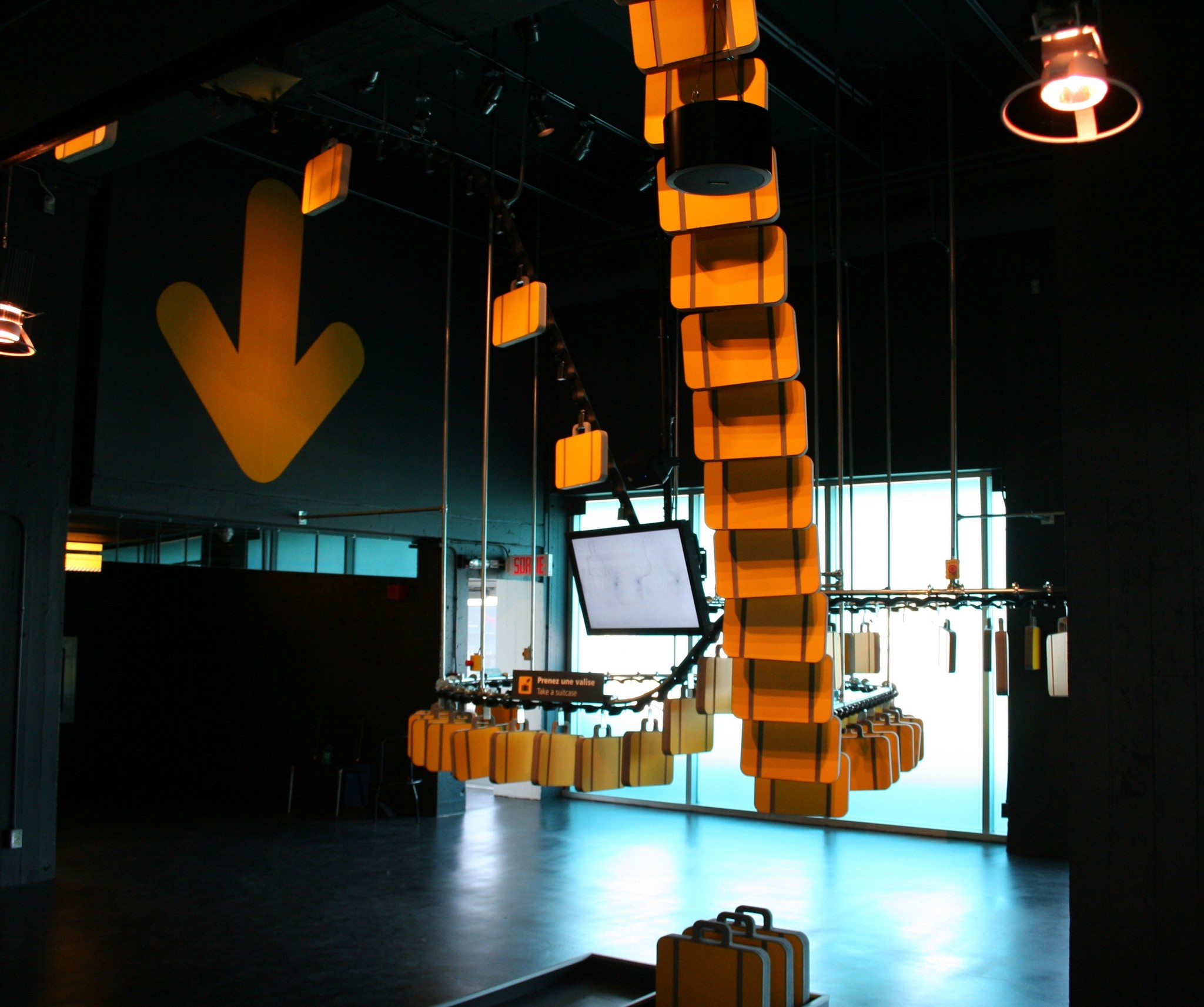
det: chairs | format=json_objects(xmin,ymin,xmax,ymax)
[{"xmin": 281, "ymin": 734, "xmax": 431, "ymax": 823}]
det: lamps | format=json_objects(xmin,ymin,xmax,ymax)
[
  {"xmin": 1002, "ymin": 1, "xmax": 1141, "ymax": 145},
  {"xmin": 271, "ymin": 41, "xmax": 657, "ymax": 211}
]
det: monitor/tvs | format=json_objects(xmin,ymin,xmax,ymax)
[{"xmin": 566, "ymin": 521, "xmax": 710, "ymax": 636}]
